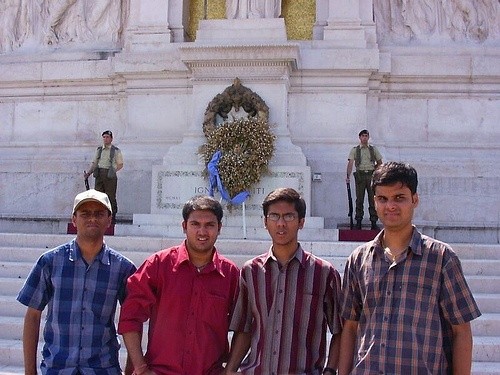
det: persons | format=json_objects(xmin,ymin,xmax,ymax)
[
  {"xmin": 221, "ymin": 187, "xmax": 343, "ymax": 375},
  {"xmin": 118, "ymin": 195, "xmax": 241, "ymax": 375},
  {"xmin": 345, "ymin": 129, "xmax": 383, "ymax": 230},
  {"xmin": 337, "ymin": 161, "xmax": 482, "ymax": 375},
  {"xmin": 84, "ymin": 130, "xmax": 124, "ymax": 224},
  {"xmin": 16, "ymin": 189, "xmax": 143, "ymax": 375}
]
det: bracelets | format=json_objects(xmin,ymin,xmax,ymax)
[
  {"xmin": 131, "ymin": 363, "xmax": 148, "ymax": 375},
  {"xmin": 323, "ymin": 367, "xmax": 337, "ymax": 375}
]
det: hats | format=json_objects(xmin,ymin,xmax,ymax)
[
  {"xmin": 73, "ymin": 188, "xmax": 113, "ymax": 216},
  {"xmin": 102, "ymin": 130, "xmax": 113, "ymax": 138},
  {"xmin": 358, "ymin": 130, "xmax": 369, "ymax": 136}
]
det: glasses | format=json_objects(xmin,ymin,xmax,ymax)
[{"xmin": 265, "ymin": 212, "xmax": 300, "ymax": 222}]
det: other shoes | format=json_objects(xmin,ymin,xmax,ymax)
[{"xmin": 112, "ymin": 213, "xmax": 117, "ymax": 224}]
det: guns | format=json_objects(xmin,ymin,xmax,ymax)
[
  {"xmin": 84, "ymin": 170, "xmax": 89, "ymax": 190},
  {"xmin": 346, "ymin": 173, "xmax": 354, "ymax": 229}
]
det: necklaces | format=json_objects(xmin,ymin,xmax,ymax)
[{"xmin": 381, "ymin": 239, "xmax": 408, "ymax": 261}]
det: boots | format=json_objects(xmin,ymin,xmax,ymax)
[
  {"xmin": 371, "ymin": 221, "xmax": 380, "ymax": 229},
  {"xmin": 352, "ymin": 221, "xmax": 361, "ymax": 229}
]
